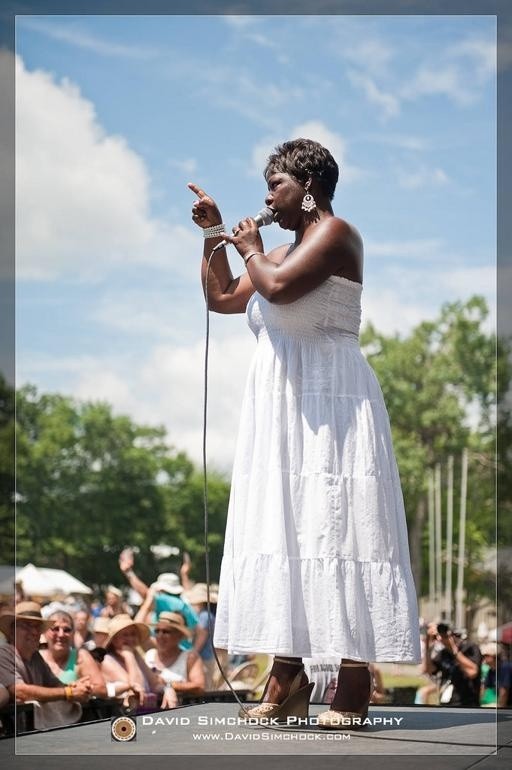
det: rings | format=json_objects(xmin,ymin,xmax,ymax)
[{"xmin": 234, "ymin": 229, "xmax": 241, "ymax": 236}]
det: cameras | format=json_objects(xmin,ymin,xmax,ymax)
[{"xmin": 429, "ymin": 620, "xmax": 454, "ymax": 638}]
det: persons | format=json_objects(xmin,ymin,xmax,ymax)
[
  {"xmin": 186, "ymin": 138, "xmax": 421, "ymax": 730},
  {"xmin": 0, "ymin": 549, "xmax": 512, "ymax": 740}
]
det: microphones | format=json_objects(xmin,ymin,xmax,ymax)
[{"xmin": 212, "ymin": 206, "xmax": 274, "ymax": 252}]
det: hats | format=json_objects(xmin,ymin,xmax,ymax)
[
  {"xmin": 0, "ymin": 601, "xmax": 57, "ymax": 642},
  {"xmin": 94, "ymin": 614, "xmax": 150, "ymax": 649},
  {"xmin": 180, "ymin": 582, "xmax": 219, "ymax": 604},
  {"xmin": 82, "ymin": 640, "xmax": 106, "ymax": 662},
  {"xmin": 148, "ymin": 611, "xmax": 191, "ymax": 638},
  {"xmin": 150, "ymin": 573, "xmax": 184, "ymax": 594}
]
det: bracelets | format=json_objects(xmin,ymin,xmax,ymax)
[
  {"xmin": 245, "ymin": 252, "xmax": 262, "ymax": 263},
  {"xmin": 201, "ymin": 223, "xmax": 225, "ymax": 239}
]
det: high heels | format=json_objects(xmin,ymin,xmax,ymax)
[
  {"xmin": 244, "ymin": 658, "xmax": 315, "ymax": 721},
  {"xmin": 317, "ymin": 663, "xmax": 373, "ymax": 729}
]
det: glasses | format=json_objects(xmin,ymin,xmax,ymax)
[
  {"xmin": 12, "ymin": 621, "xmax": 43, "ymax": 632},
  {"xmin": 50, "ymin": 625, "xmax": 72, "ymax": 632},
  {"xmin": 154, "ymin": 628, "xmax": 170, "ymax": 634},
  {"xmin": 95, "ymin": 650, "xmax": 104, "ymax": 663}
]
